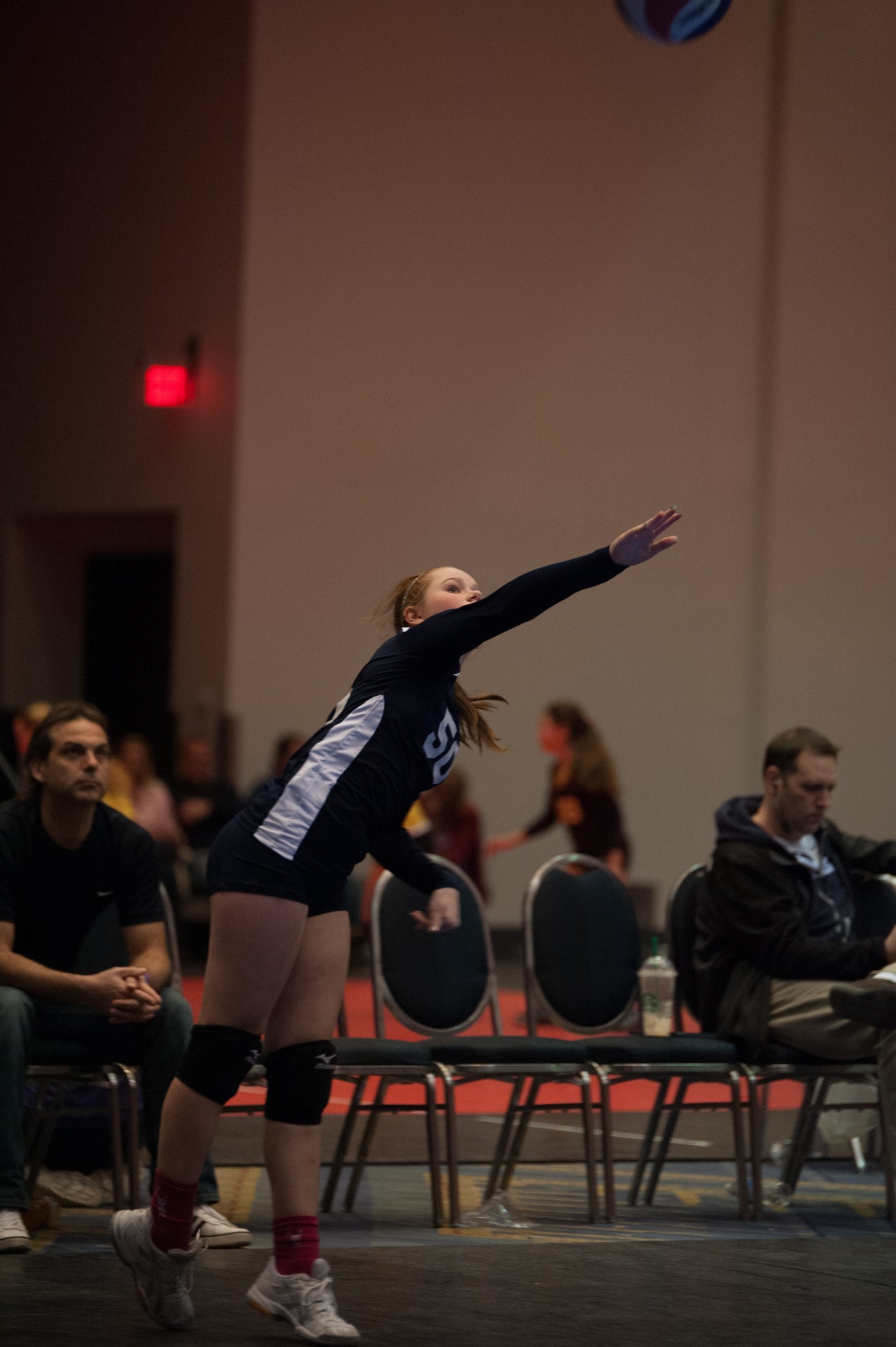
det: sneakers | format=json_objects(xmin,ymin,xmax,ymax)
[
  {"xmin": 243, "ymin": 1254, "xmax": 362, "ymax": 1344},
  {"xmin": 111, "ymin": 1206, "xmax": 204, "ymax": 1330},
  {"xmin": 0, "ymin": 1208, "xmax": 32, "ymax": 1252},
  {"xmin": 191, "ymin": 1204, "xmax": 253, "ymax": 1247}
]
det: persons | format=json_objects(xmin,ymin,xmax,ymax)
[
  {"xmin": 108, "ymin": 507, "xmax": 683, "ymax": 1344},
  {"xmin": 481, "ymin": 699, "xmax": 631, "ymax": 1024},
  {"xmin": 0, "ymin": 696, "xmax": 308, "ymax": 1249},
  {"xmin": 688, "ymin": 723, "xmax": 896, "ymax": 1216},
  {"xmin": 406, "ymin": 767, "xmax": 491, "ymax": 905}
]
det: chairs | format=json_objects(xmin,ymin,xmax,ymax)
[
  {"xmin": 217, "ymin": 1053, "xmax": 271, "ymax": 1122},
  {"xmin": 321, "ymin": 920, "xmax": 445, "ymax": 1231},
  {"xmin": 642, "ymin": 858, "xmax": 896, "ymax": 1223},
  {"xmin": 25, "ymin": 881, "xmax": 183, "ymax": 1223},
  {"xmin": 499, "ymin": 852, "xmax": 756, "ymax": 1226},
  {"xmin": 369, "ymin": 849, "xmax": 603, "ymax": 1228}
]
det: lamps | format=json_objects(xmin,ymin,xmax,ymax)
[{"xmin": 140, "ymin": 332, "xmax": 202, "ymax": 412}]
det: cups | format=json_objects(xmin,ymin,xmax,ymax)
[{"xmin": 637, "ymin": 956, "xmax": 678, "ymax": 1036}]
